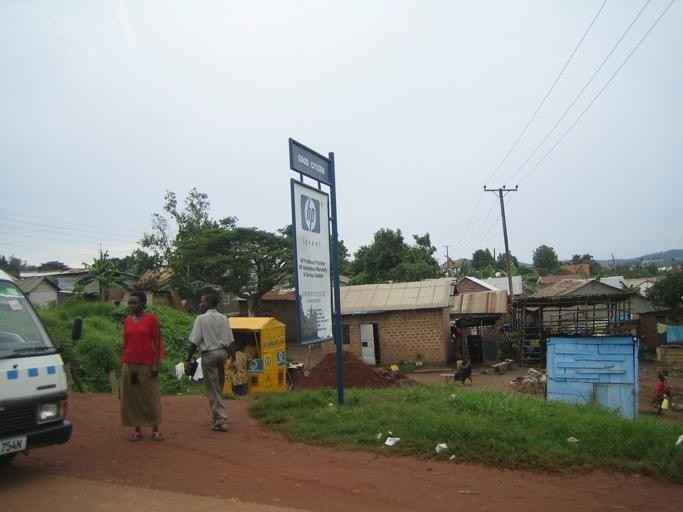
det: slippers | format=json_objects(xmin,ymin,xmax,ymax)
[
  {"xmin": 129, "ymin": 432, "xmax": 141, "ymax": 441},
  {"xmin": 152, "ymin": 431, "xmax": 164, "ymax": 441}
]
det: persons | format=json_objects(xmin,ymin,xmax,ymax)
[
  {"xmin": 453, "ymin": 349, "xmax": 464, "ymax": 369},
  {"xmin": 452, "ymin": 354, "xmax": 472, "ymax": 386},
  {"xmin": 183, "ymin": 292, "xmax": 236, "ymax": 431},
  {"xmin": 651, "ymin": 371, "xmax": 665, "ymax": 414},
  {"xmin": 662, "ymin": 368, "xmax": 670, "ymax": 398},
  {"xmin": 119, "ymin": 291, "xmax": 164, "ymax": 441}
]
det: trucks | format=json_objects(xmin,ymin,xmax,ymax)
[{"xmin": 0, "ymin": 269, "xmax": 83, "ymax": 464}]
